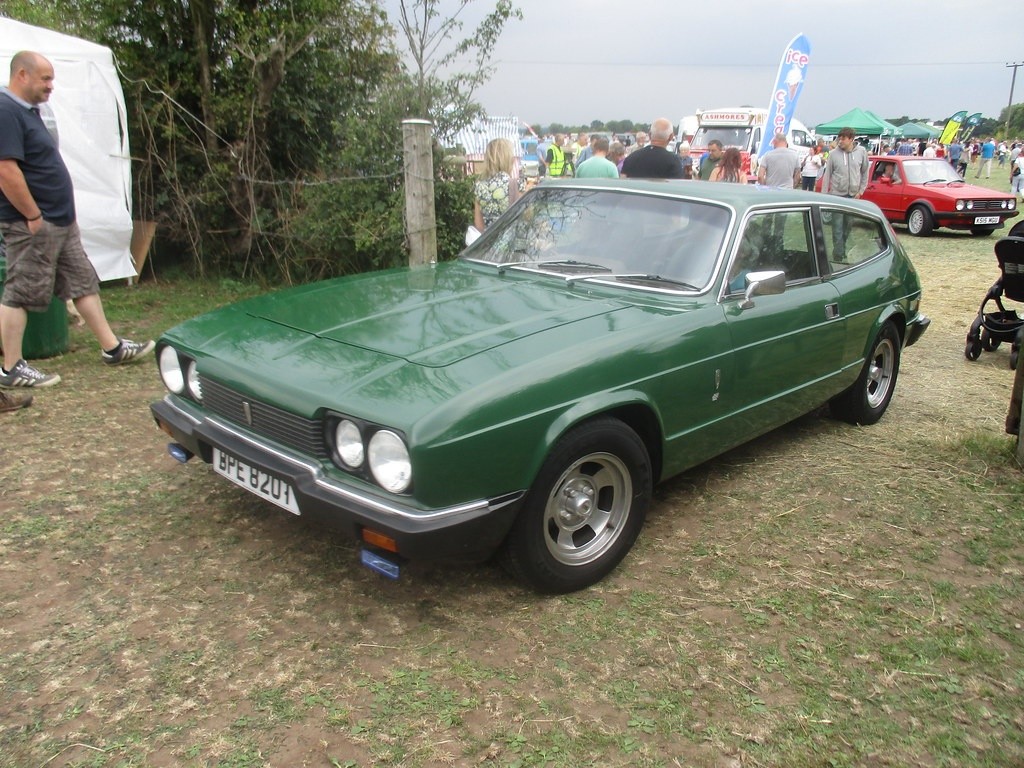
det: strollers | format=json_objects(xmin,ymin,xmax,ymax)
[{"xmin": 964, "ymin": 220, "xmax": 1024, "ymax": 370}]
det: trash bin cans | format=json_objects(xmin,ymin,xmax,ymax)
[{"xmin": 0, "ymin": 220, "xmax": 71, "ymax": 360}]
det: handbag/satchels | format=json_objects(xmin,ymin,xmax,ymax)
[{"xmin": 1013, "ymin": 166, "xmax": 1021, "ymax": 176}]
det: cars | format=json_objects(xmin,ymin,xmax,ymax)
[
  {"xmin": 518, "ymin": 139, "xmax": 554, "ymax": 176},
  {"xmin": 813, "ymin": 155, "xmax": 1020, "ymax": 236},
  {"xmin": 569, "ymin": 132, "xmax": 679, "ymax": 156},
  {"xmin": 149, "ymin": 178, "xmax": 932, "ymax": 597}
]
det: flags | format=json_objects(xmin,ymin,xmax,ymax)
[{"xmin": 758, "ymin": 33, "xmax": 811, "ymax": 157}]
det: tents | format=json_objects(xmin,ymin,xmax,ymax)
[
  {"xmin": 0, "ymin": 17, "xmax": 138, "ymax": 286},
  {"xmin": 815, "ymin": 107, "xmax": 941, "ymax": 156}
]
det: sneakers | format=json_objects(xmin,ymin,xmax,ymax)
[
  {"xmin": 0, "ymin": 391, "xmax": 34, "ymax": 411},
  {"xmin": 0, "ymin": 359, "xmax": 61, "ymax": 388},
  {"xmin": 102, "ymin": 339, "xmax": 157, "ymax": 367}
]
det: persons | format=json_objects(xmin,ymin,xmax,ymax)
[
  {"xmin": 474, "ymin": 137, "xmax": 538, "ymax": 265},
  {"xmin": 1008, "ymin": 137, "xmax": 1024, "ymax": 203},
  {"xmin": 759, "ymin": 134, "xmax": 801, "ymax": 236},
  {"xmin": 880, "ymin": 137, "xmax": 945, "ymax": 158},
  {"xmin": 700, "ymin": 140, "xmax": 747, "ymax": 184},
  {"xmin": 801, "ymin": 138, "xmax": 828, "ymax": 191},
  {"xmin": 821, "ymin": 128, "xmax": 869, "ymax": 262},
  {"xmin": 947, "ymin": 138, "xmax": 1008, "ymax": 179},
  {"xmin": 0, "ymin": 389, "xmax": 31, "ymax": 412},
  {"xmin": 713, "ymin": 230, "xmax": 762, "ymax": 293},
  {"xmin": 876, "ymin": 164, "xmax": 902, "ymax": 185},
  {"xmin": 536, "ymin": 118, "xmax": 692, "ymax": 178},
  {"xmin": 0, "ymin": 51, "xmax": 156, "ymax": 387}
]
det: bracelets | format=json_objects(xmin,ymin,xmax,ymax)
[{"xmin": 27, "ymin": 213, "xmax": 42, "ymax": 222}]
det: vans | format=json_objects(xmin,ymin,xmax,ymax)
[{"xmin": 679, "ymin": 107, "xmax": 820, "ymax": 185}]
